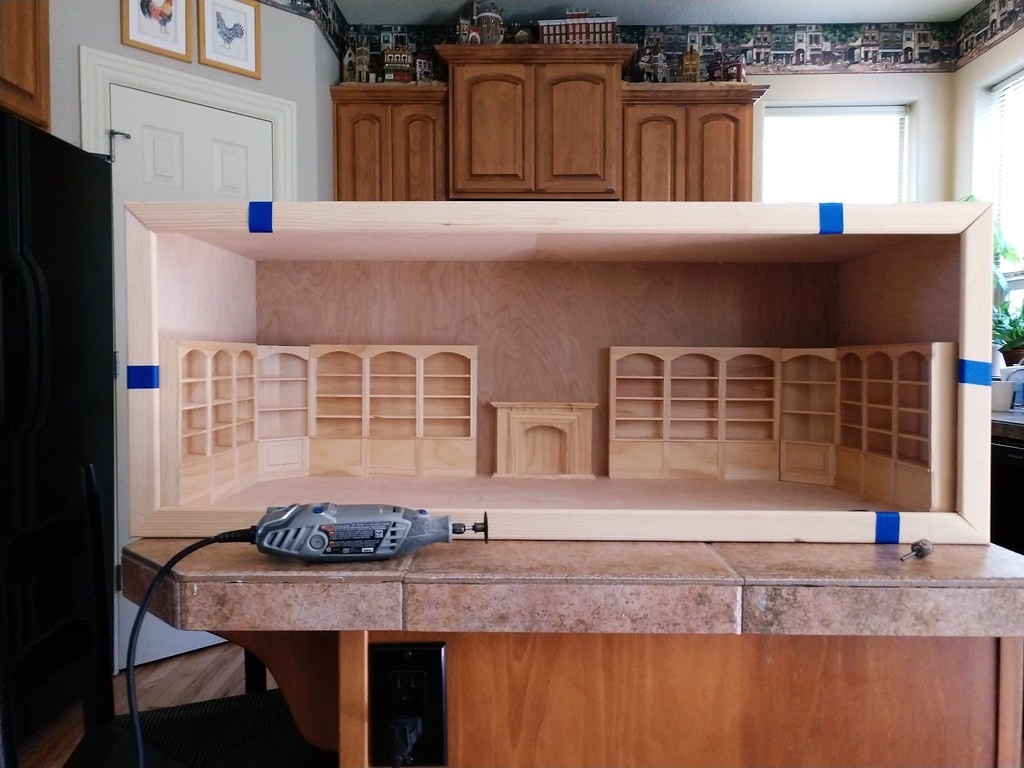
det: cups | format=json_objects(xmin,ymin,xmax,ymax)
[
  {"xmin": 1000, "ymin": 364, "xmax": 1024, "ymax": 405},
  {"xmin": 991, "ymin": 380, "xmax": 1014, "ymax": 412}
]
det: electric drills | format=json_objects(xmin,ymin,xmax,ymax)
[{"xmin": 254, "ymin": 502, "xmax": 490, "ymax": 566}]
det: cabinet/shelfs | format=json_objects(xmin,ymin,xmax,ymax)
[
  {"xmin": 623, "ymin": 81, "xmax": 769, "ymax": 204},
  {"xmin": 778, "ymin": 349, "xmax": 836, "ymax": 482},
  {"xmin": 0, "ymin": 0, "xmax": 50, "ymax": 132},
  {"xmin": 160, "ymin": 342, "xmax": 255, "ymax": 506},
  {"xmin": 255, "ymin": 347, "xmax": 309, "ymax": 482},
  {"xmin": 333, "ymin": 86, "xmax": 448, "ymax": 202},
  {"xmin": 837, "ymin": 341, "xmax": 956, "ymax": 514},
  {"xmin": 608, "ymin": 345, "xmax": 776, "ymax": 475},
  {"xmin": 437, "ymin": 44, "xmax": 636, "ymax": 204}
]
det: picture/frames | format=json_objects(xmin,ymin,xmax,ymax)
[
  {"xmin": 120, "ymin": 0, "xmax": 191, "ymax": 61},
  {"xmin": 197, "ymin": 0, "xmax": 261, "ymax": 80}
]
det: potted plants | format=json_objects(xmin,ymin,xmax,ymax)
[
  {"xmin": 993, "ymin": 272, "xmax": 1024, "ymax": 366},
  {"xmin": 310, "ymin": 344, "xmax": 477, "ymax": 481}
]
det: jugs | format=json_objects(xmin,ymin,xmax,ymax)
[{"xmin": 992, "ymin": 345, "xmax": 1006, "ymax": 377}]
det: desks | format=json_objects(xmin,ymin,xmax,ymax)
[
  {"xmin": 491, "ymin": 401, "xmax": 601, "ymax": 481},
  {"xmin": 123, "ymin": 539, "xmax": 1024, "ymax": 768}
]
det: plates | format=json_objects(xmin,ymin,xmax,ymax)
[{"xmin": 991, "ymin": 376, "xmax": 1002, "ymax": 379}]
